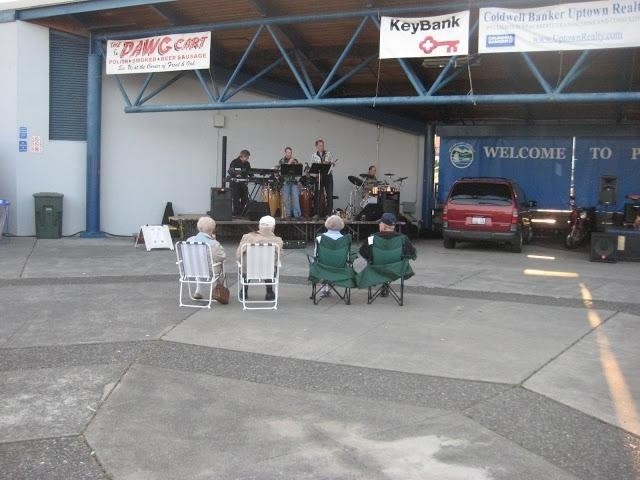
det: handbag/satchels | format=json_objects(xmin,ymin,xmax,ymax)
[{"xmin": 212, "ymin": 284, "xmax": 229, "ymax": 304}]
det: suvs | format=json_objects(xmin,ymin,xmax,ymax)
[{"xmin": 438, "ymin": 178, "xmax": 537, "ymax": 252}]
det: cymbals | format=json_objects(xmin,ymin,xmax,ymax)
[
  {"xmin": 384, "ymin": 173, "xmax": 395, "ymax": 176},
  {"xmin": 360, "ymin": 174, "xmax": 375, "ymax": 179},
  {"xmin": 393, "ymin": 177, "xmax": 408, "ymax": 181},
  {"xmin": 347, "ymin": 175, "xmax": 363, "ymax": 187}
]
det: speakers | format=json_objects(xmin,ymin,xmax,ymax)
[
  {"xmin": 589, "ymin": 230, "xmax": 618, "ymax": 263},
  {"xmin": 210, "ymin": 187, "xmax": 233, "ymax": 222},
  {"xmin": 377, "ymin": 190, "xmax": 400, "ymax": 220},
  {"xmin": 246, "ymin": 199, "xmax": 271, "ymax": 220},
  {"xmin": 598, "ymin": 175, "xmax": 617, "ymax": 202},
  {"xmin": 354, "ymin": 203, "xmax": 377, "ymax": 221}
]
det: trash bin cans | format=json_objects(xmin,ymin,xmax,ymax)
[{"xmin": 33, "ymin": 192, "xmax": 64, "ymax": 239}]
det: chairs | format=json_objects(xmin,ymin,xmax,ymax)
[
  {"xmin": 236, "ymin": 242, "xmax": 281, "ymax": 310},
  {"xmin": 305, "ymin": 233, "xmax": 358, "ymax": 306},
  {"xmin": 174, "ymin": 240, "xmax": 227, "ymax": 310},
  {"xmin": 356, "ymin": 234, "xmax": 415, "ymax": 307}
]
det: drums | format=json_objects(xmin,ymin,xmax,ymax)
[
  {"xmin": 360, "ymin": 193, "xmax": 378, "ymax": 209},
  {"xmin": 382, "ymin": 185, "xmax": 395, "ymax": 192},
  {"xmin": 370, "ymin": 185, "xmax": 382, "ymax": 196}
]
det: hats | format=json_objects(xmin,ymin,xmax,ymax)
[
  {"xmin": 376, "ymin": 213, "xmax": 397, "ymax": 226},
  {"xmin": 259, "ymin": 216, "xmax": 276, "ymax": 228}
]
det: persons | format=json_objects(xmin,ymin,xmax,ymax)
[
  {"xmin": 280, "ymin": 147, "xmax": 303, "ymax": 220},
  {"xmin": 362, "ymin": 166, "xmax": 378, "ymax": 198},
  {"xmin": 300, "ymin": 168, "xmax": 317, "ymax": 199},
  {"xmin": 359, "ymin": 212, "xmax": 416, "ymax": 298},
  {"xmin": 230, "ymin": 150, "xmax": 254, "ymax": 219},
  {"xmin": 237, "ymin": 216, "xmax": 283, "ymax": 301},
  {"xmin": 187, "ymin": 216, "xmax": 227, "ymax": 300},
  {"xmin": 312, "ymin": 140, "xmax": 333, "ymax": 218},
  {"xmin": 314, "ymin": 216, "xmax": 345, "ymax": 297}
]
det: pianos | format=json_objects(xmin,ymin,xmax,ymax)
[{"xmin": 223, "ymin": 176, "xmax": 271, "ymax": 184}]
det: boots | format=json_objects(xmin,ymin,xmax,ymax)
[
  {"xmin": 265, "ymin": 287, "xmax": 275, "ymax": 300},
  {"xmin": 240, "ymin": 287, "xmax": 248, "ymax": 299}
]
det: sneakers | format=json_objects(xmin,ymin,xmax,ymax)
[{"xmin": 193, "ymin": 293, "xmax": 202, "ymax": 299}]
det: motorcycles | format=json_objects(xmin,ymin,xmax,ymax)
[{"xmin": 564, "ymin": 204, "xmax": 597, "ymax": 247}]
